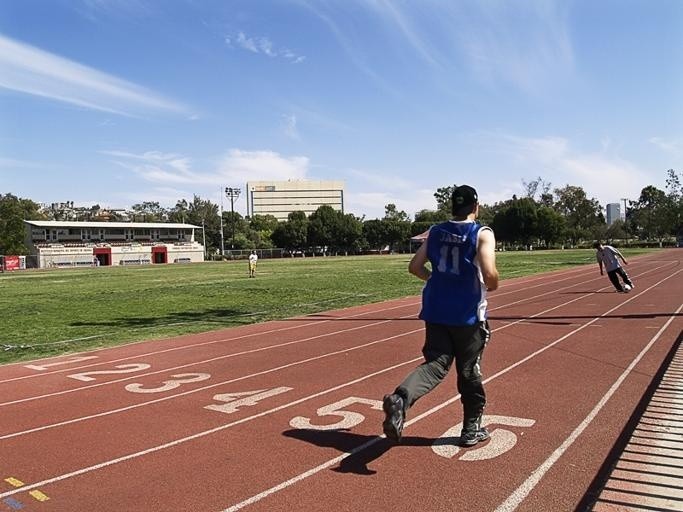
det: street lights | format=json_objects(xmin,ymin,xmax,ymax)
[
  {"xmin": 225, "ymin": 186, "xmax": 242, "ymax": 249},
  {"xmin": 621, "ymin": 198, "xmax": 629, "ymax": 246}
]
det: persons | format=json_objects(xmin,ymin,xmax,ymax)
[
  {"xmin": 248, "ymin": 249, "xmax": 258, "ymax": 278},
  {"xmin": 593, "ymin": 240, "xmax": 636, "ymax": 293},
  {"xmin": 382, "ymin": 183, "xmax": 500, "ymax": 446}
]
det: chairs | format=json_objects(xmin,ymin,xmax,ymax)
[{"xmin": 32, "ymin": 239, "xmax": 194, "ymax": 248}]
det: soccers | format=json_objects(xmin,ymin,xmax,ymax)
[{"xmin": 622, "ymin": 284, "xmax": 631, "ymax": 292}]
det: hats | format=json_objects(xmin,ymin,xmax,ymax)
[{"xmin": 453, "ymin": 186, "xmax": 477, "ymax": 216}]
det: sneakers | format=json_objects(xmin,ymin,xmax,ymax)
[
  {"xmin": 460, "ymin": 427, "xmax": 489, "ymax": 446},
  {"xmin": 383, "ymin": 394, "xmax": 404, "ymax": 444}
]
